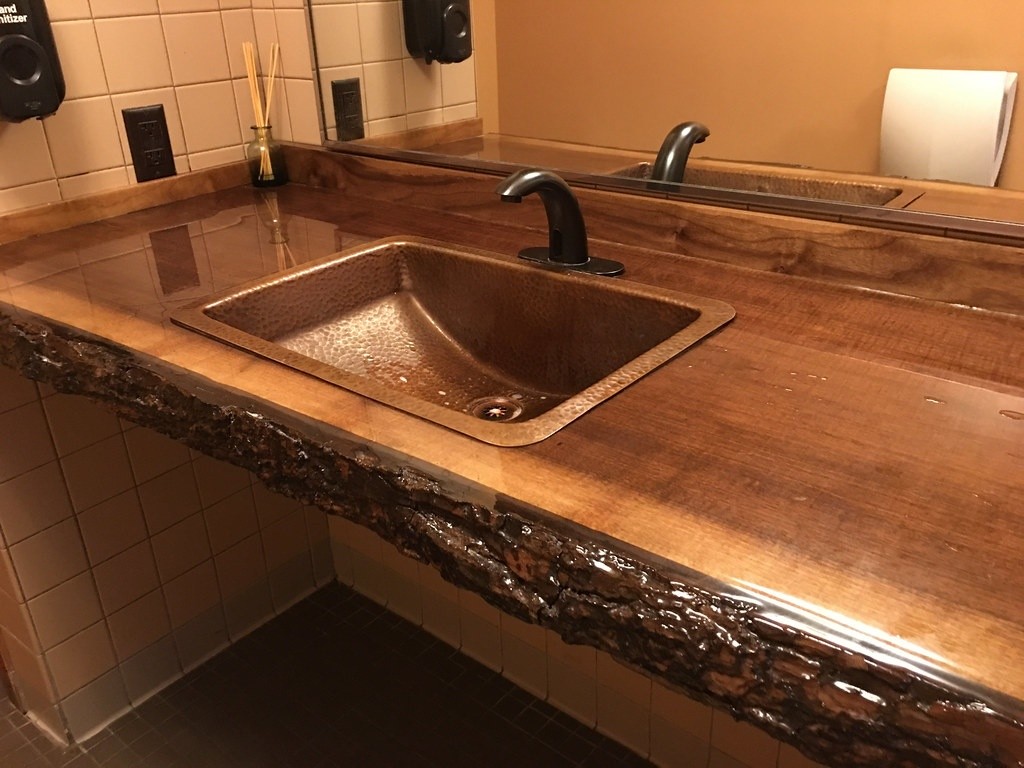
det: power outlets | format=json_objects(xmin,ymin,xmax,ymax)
[
  {"xmin": 330, "ymin": 77, "xmax": 367, "ymax": 142},
  {"xmin": 123, "ymin": 104, "xmax": 178, "ymax": 183}
]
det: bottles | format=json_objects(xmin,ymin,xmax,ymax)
[{"xmin": 248, "ymin": 124, "xmax": 288, "ymax": 187}]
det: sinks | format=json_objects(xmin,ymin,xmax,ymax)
[
  {"xmin": 168, "ymin": 234, "xmax": 738, "ymax": 450},
  {"xmin": 595, "ymin": 159, "xmax": 927, "ymax": 218}
]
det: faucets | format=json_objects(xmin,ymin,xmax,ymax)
[
  {"xmin": 499, "ymin": 170, "xmax": 625, "ymax": 277},
  {"xmin": 644, "ymin": 121, "xmax": 710, "ymax": 193}
]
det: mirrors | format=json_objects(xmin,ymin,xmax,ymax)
[{"xmin": 305, "ymin": 0, "xmax": 1024, "ymax": 246}]
